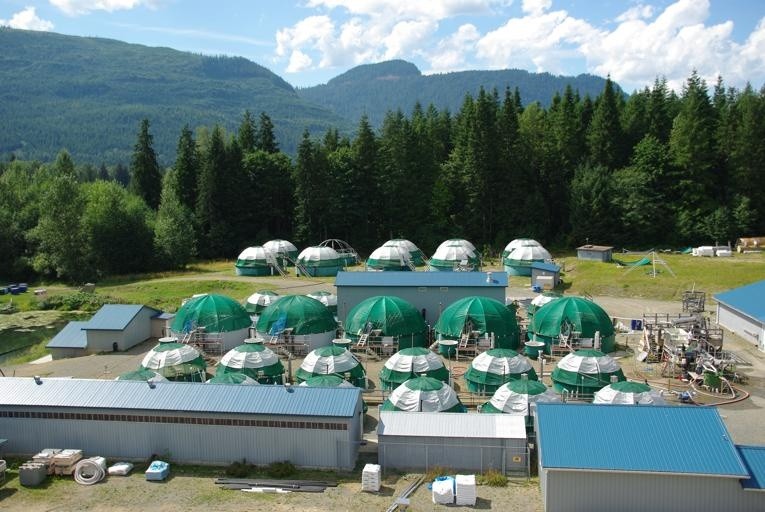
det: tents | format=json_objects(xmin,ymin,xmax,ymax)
[
  {"xmin": 116, "ymin": 334, "xmax": 628, "ymax": 402},
  {"xmin": 165, "ymin": 293, "xmax": 617, "ymax": 356},
  {"xmin": 378, "ymin": 377, "xmax": 666, "ymax": 414},
  {"xmin": 235, "ymin": 236, "xmax": 553, "ymax": 277}
]
